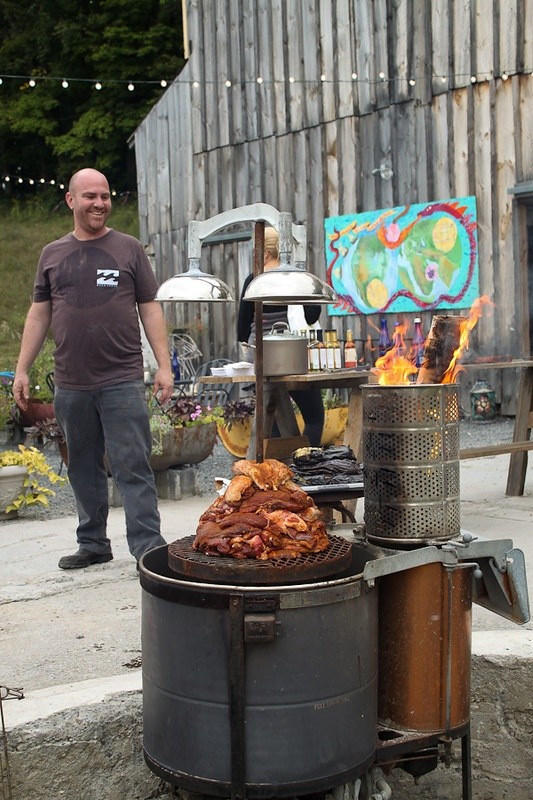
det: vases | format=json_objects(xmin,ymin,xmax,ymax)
[{"xmin": 152, "ymin": 423, "xmax": 217, "ymax": 472}]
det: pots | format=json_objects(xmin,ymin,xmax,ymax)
[{"xmin": 241, "ymin": 321, "xmax": 320, "ymax": 376}]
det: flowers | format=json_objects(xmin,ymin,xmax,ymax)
[{"xmin": 149, "ymin": 404, "xmax": 225, "ymax": 454}]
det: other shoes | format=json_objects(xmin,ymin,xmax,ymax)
[{"xmin": 58, "ymin": 548, "xmax": 114, "ymax": 568}]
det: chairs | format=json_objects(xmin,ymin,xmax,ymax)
[{"xmin": 192, "ymin": 359, "xmax": 234, "ymax": 410}]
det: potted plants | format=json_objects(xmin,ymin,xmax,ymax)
[
  {"xmin": 23, "ymin": 419, "xmax": 69, "ymax": 468},
  {"xmin": 0, "ymin": 444, "xmax": 68, "ymax": 521},
  {"xmin": 0, "ymin": 385, "xmax": 15, "ymax": 446},
  {"xmin": 21, "ymin": 372, "xmax": 55, "ymax": 426}
]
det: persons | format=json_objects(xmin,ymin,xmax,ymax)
[
  {"xmin": 236, "ymin": 227, "xmax": 323, "ymax": 437},
  {"xmin": 12, "ymin": 168, "xmax": 174, "ymax": 571}
]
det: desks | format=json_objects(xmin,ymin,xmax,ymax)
[
  {"xmin": 146, "ymin": 381, "xmax": 192, "ymax": 415},
  {"xmin": 200, "ymin": 358, "xmax": 533, "ymax": 522}
]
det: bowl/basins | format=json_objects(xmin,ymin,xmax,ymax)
[{"xmin": 209, "ymin": 366, "xmax": 225, "ymax": 376}]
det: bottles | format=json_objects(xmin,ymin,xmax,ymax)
[
  {"xmin": 171, "ymin": 351, "xmax": 180, "ymax": 380},
  {"xmin": 378, "ymin": 318, "xmax": 392, "ymax": 358},
  {"xmin": 344, "ymin": 329, "xmax": 357, "ymax": 368},
  {"xmin": 293, "ymin": 328, "xmax": 342, "ymax": 373},
  {"xmin": 144, "ymin": 360, "xmax": 150, "ymax": 383},
  {"xmin": 410, "ymin": 318, "xmax": 426, "ymax": 368},
  {"xmin": 393, "ymin": 322, "xmax": 407, "ymax": 359}
]
